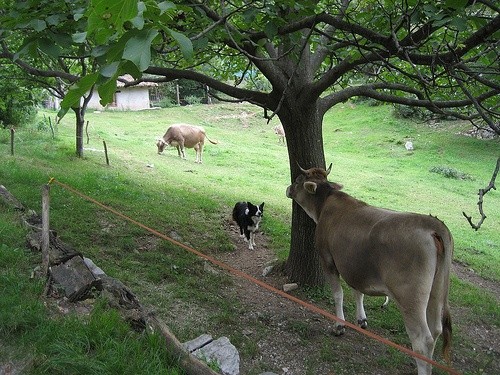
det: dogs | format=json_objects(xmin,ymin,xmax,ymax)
[
  {"xmin": 233, "ymin": 201, "xmax": 265, "ymax": 250},
  {"xmin": 273, "ymin": 125, "xmax": 285, "ymax": 143}
]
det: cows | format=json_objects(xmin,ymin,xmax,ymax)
[
  {"xmin": 286, "ymin": 162, "xmax": 455, "ymax": 375},
  {"xmin": 156, "ymin": 124, "xmax": 218, "ymax": 164}
]
